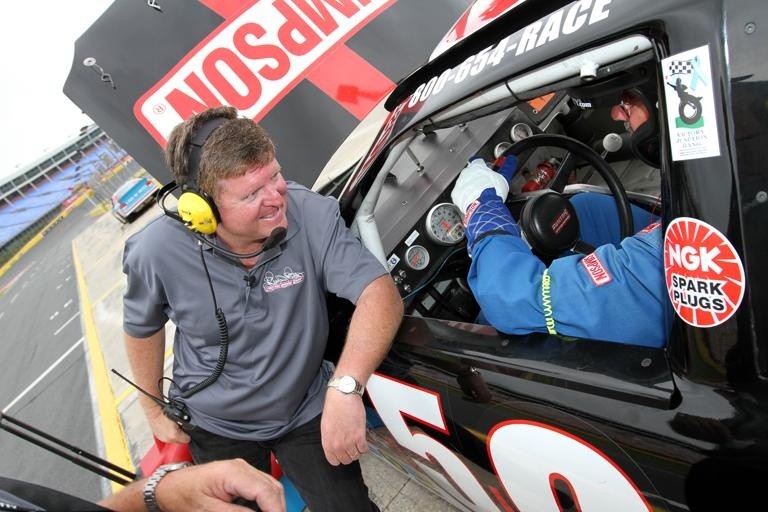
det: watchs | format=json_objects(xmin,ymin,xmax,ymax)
[
  {"xmin": 142, "ymin": 459, "xmax": 189, "ymax": 512},
  {"xmin": 325, "ymin": 375, "xmax": 368, "ymax": 399}
]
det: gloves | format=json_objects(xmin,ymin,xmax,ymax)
[{"xmin": 450, "ymin": 158, "xmax": 509, "ymax": 215}]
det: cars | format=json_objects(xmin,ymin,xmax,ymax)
[
  {"xmin": 111, "ymin": 176, "xmax": 160, "ymax": 224},
  {"xmin": 62, "ymin": 0, "xmax": 768, "ymax": 511}
]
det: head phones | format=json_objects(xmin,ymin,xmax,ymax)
[{"xmin": 176, "ymin": 117, "xmax": 231, "ymax": 239}]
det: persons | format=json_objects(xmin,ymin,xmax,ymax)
[
  {"xmin": 97, "ymin": 456, "xmax": 289, "ymax": 510},
  {"xmin": 121, "ymin": 106, "xmax": 405, "ymax": 511},
  {"xmin": 450, "ymin": 78, "xmax": 669, "ymax": 348}
]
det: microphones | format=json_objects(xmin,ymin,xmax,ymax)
[{"xmin": 190, "ymin": 226, "xmax": 287, "ymax": 259}]
